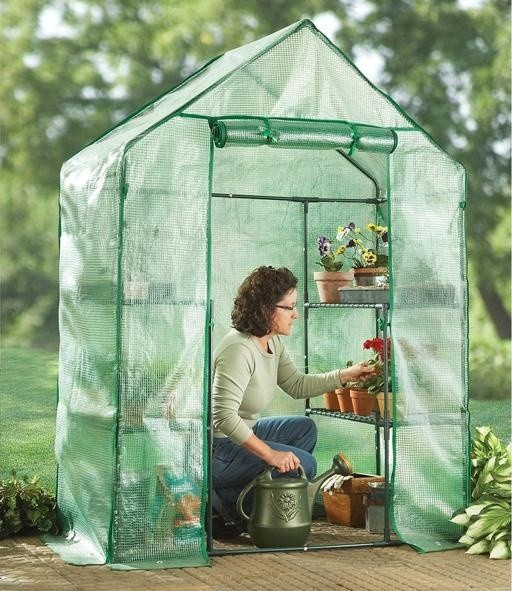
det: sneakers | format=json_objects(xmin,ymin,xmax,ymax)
[{"xmin": 204, "ymin": 502, "xmax": 240, "ymax": 541}]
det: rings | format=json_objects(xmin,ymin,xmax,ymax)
[{"xmin": 285, "ymin": 464, "xmax": 290, "ymax": 468}]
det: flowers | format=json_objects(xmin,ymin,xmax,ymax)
[
  {"xmin": 314, "ymin": 234, "xmax": 347, "ymax": 273},
  {"xmin": 340, "ymin": 336, "xmax": 393, "ymax": 391},
  {"xmin": 336, "ymin": 222, "xmax": 389, "ymax": 269}
]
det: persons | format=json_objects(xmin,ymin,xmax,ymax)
[{"xmin": 199, "ymin": 263, "xmax": 383, "ymax": 541}]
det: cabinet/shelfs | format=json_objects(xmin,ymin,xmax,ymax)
[{"xmin": 305, "ymin": 201, "xmax": 396, "ymax": 543}]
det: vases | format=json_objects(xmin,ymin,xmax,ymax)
[
  {"xmin": 321, "ymin": 473, "xmax": 383, "ymax": 528},
  {"xmin": 353, "ymin": 267, "xmax": 389, "ymax": 289},
  {"xmin": 376, "ymin": 391, "xmax": 405, "ymax": 419},
  {"xmin": 349, "ymin": 388, "xmax": 375, "ymax": 415},
  {"xmin": 313, "ymin": 272, "xmax": 355, "ymax": 303},
  {"xmin": 334, "ymin": 388, "xmax": 355, "ymax": 412},
  {"xmin": 322, "ymin": 390, "xmax": 341, "ymax": 411}
]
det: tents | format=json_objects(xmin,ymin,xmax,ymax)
[{"xmin": 40, "ymin": 18, "xmax": 474, "ymax": 576}]
